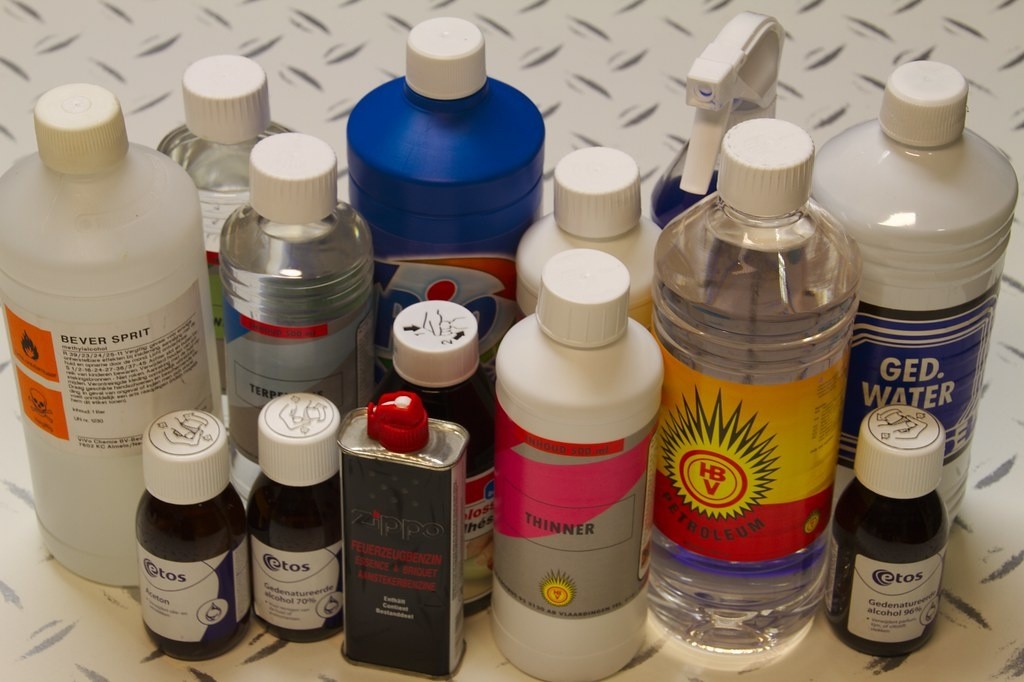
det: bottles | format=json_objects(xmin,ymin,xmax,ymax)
[
  {"xmin": 488, "ymin": 245, "xmax": 669, "ymax": 682},
  {"xmin": 809, "ymin": 58, "xmax": 1020, "ymax": 524},
  {"xmin": 514, "ymin": 145, "xmax": 662, "ymax": 335},
  {"xmin": 245, "ymin": 390, "xmax": 343, "ymax": 644},
  {"xmin": 650, "ymin": 115, "xmax": 863, "ymax": 674},
  {"xmin": 217, "ymin": 130, "xmax": 376, "ymax": 510},
  {"xmin": 372, "ymin": 300, "xmax": 496, "ymax": 616},
  {"xmin": 823, "ymin": 403, "xmax": 949, "ymax": 659},
  {"xmin": 152, "ymin": 53, "xmax": 298, "ymax": 392},
  {"xmin": 0, "ymin": 81, "xmax": 221, "ymax": 587},
  {"xmin": 133, "ymin": 407, "xmax": 249, "ymax": 663},
  {"xmin": 342, "ymin": 14, "xmax": 547, "ymax": 418}
]
